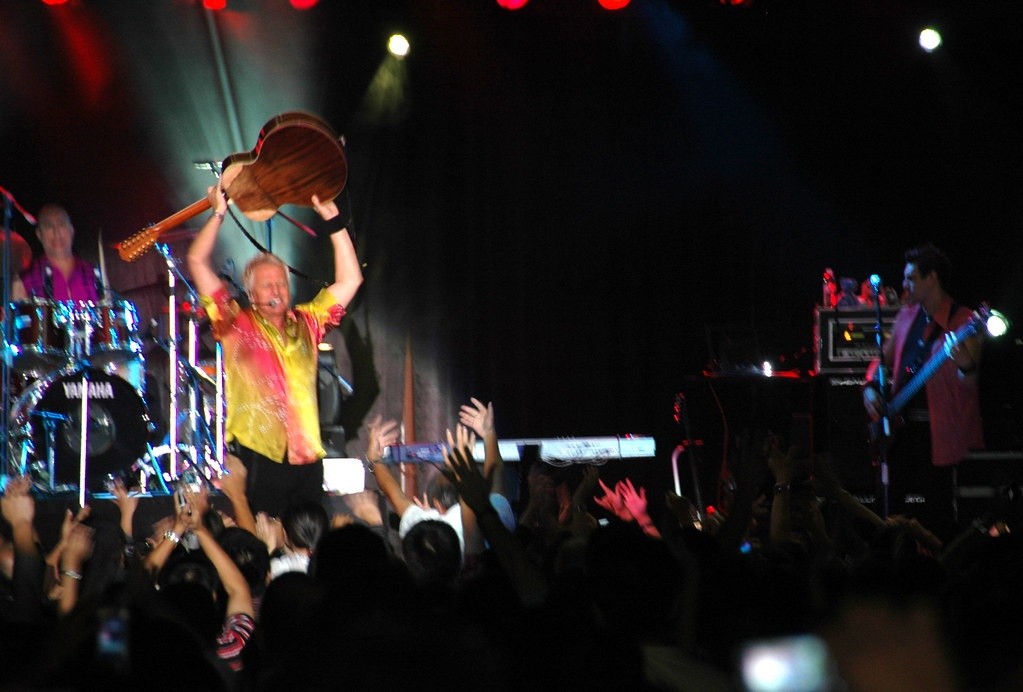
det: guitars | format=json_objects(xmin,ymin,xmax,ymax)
[
  {"xmin": 856, "ymin": 305, "xmax": 992, "ymax": 477},
  {"xmin": 117, "ymin": 111, "xmax": 350, "ymax": 264}
]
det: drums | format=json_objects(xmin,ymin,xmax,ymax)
[
  {"xmin": 10, "ymin": 366, "xmax": 151, "ymax": 492},
  {"xmin": 6, "ymin": 297, "xmax": 142, "ymax": 372}
]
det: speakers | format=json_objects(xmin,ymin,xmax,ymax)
[{"xmin": 815, "ymin": 371, "xmax": 944, "ymax": 526}]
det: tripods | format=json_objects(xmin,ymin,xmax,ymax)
[{"xmin": 127, "ymin": 239, "xmax": 228, "ymax": 494}]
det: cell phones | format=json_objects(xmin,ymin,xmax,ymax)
[
  {"xmin": 97, "ymin": 608, "xmax": 128, "ymax": 657},
  {"xmin": 741, "ymin": 635, "xmax": 835, "ymax": 692}
]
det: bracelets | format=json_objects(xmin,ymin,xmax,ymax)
[
  {"xmin": 163, "ymin": 530, "xmax": 180, "ymax": 547},
  {"xmin": 60, "ymin": 569, "xmax": 83, "ymax": 580},
  {"xmin": 368, "ymin": 460, "xmax": 379, "ymax": 472},
  {"xmin": 325, "ymin": 214, "xmax": 346, "ymax": 235},
  {"xmin": 208, "ymin": 212, "xmax": 223, "ymax": 223}
]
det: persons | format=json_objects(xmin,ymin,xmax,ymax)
[
  {"xmin": 13, "ymin": 204, "xmax": 101, "ymax": 302},
  {"xmin": 185, "ymin": 175, "xmax": 364, "ymax": 523},
  {"xmin": 863, "ymin": 248, "xmax": 982, "ymax": 483},
  {"xmin": 0, "ymin": 399, "xmax": 1023, "ymax": 692}
]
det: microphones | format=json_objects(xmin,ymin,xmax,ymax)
[
  {"xmin": 94, "ymin": 266, "xmax": 105, "ymax": 298},
  {"xmin": 871, "ymin": 275, "xmax": 882, "ymax": 291},
  {"xmin": 44, "ymin": 267, "xmax": 53, "ymax": 299},
  {"xmin": 139, "ymin": 313, "xmax": 160, "ymax": 342},
  {"xmin": 254, "ymin": 301, "xmax": 276, "ymax": 308}
]
